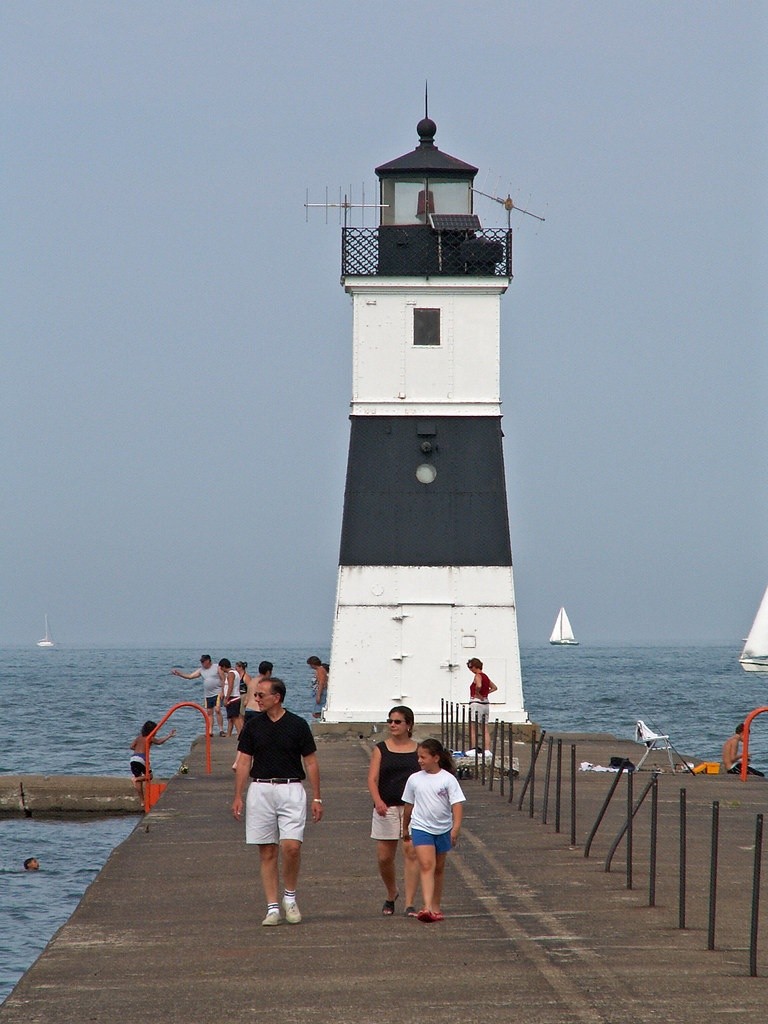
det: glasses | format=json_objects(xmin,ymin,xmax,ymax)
[
  {"xmin": 387, "ymin": 719, "xmax": 406, "ymax": 724},
  {"xmin": 469, "ymin": 665, "xmax": 474, "ymax": 668},
  {"xmin": 201, "ymin": 660, "xmax": 205, "ymax": 663},
  {"xmin": 254, "ymin": 692, "xmax": 275, "ymax": 698}
]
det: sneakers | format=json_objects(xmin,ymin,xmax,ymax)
[
  {"xmin": 282, "ymin": 897, "xmax": 301, "ymax": 924},
  {"xmin": 261, "ymin": 911, "xmax": 282, "ymax": 926}
]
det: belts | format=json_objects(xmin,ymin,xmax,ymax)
[{"xmin": 253, "ymin": 778, "xmax": 301, "ymax": 784}]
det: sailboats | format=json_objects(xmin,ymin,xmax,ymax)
[
  {"xmin": 549, "ymin": 607, "xmax": 580, "ymax": 645},
  {"xmin": 738, "ymin": 584, "xmax": 768, "ymax": 672},
  {"xmin": 36, "ymin": 614, "xmax": 55, "ymax": 647}
]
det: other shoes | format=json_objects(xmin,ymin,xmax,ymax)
[
  {"xmin": 205, "ymin": 733, "xmax": 214, "ymax": 737},
  {"xmin": 485, "ymin": 750, "xmax": 492, "ymax": 756},
  {"xmin": 220, "ymin": 731, "xmax": 226, "ymax": 737},
  {"xmin": 466, "ymin": 749, "xmax": 475, "ymax": 756}
]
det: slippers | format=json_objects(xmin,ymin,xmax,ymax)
[
  {"xmin": 383, "ymin": 894, "xmax": 399, "ymax": 914},
  {"xmin": 417, "ymin": 909, "xmax": 444, "ymax": 923},
  {"xmin": 402, "ymin": 907, "xmax": 418, "ymax": 917}
]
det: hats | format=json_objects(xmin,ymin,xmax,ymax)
[{"xmin": 199, "ymin": 655, "xmax": 210, "ymax": 661}]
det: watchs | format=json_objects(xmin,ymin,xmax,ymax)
[{"xmin": 313, "ymin": 798, "xmax": 323, "ymax": 803}]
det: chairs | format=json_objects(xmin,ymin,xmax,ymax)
[{"xmin": 634, "ymin": 720, "xmax": 676, "ymax": 774}]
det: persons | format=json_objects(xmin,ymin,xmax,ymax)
[
  {"xmin": 306, "ymin": 656, "xmax": 330, "ymax": 717},
  {"xmin": 367, "ymin": 705, "xmax": 421, "ymax": 918},
  {"xmin": 723, "ymin": 723, "xmax": 764, "ymax": 777},
  {"xmin": 230, "ymin": 661, "xmax": 273, "ymax": 770},
  {"xmin": 129, "ymin": 721, "xmax": 176, "ymax": 807},
  {"xmin": 171, "ymin": 655, "xmax": 226, "ymax": 737},
  {"xmin": 231, "ymin": 677, "xmax": 323, "ymax": 926},
  {"xmin": 402, "ymin": 738, "xmax": 462, "ymax": 922},
  {"xmin": 218, "ymin": 658, "xmax": 252, "ymax": 738},
  {"xmin": 465, "ymin": 658, "xmax": 497, "ymax": 756},
  {"xmin": 24, "ymin": 857, "xmax": 39, "ymax": 872}
]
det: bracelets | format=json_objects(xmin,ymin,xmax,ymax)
[{"xmin": 225, "ymin": 697, "xmax": 229, "ymax": 700}]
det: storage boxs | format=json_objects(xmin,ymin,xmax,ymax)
[{"xmin": 692, "ymin": 762, "xmax": 721, "ymax": 775}]
